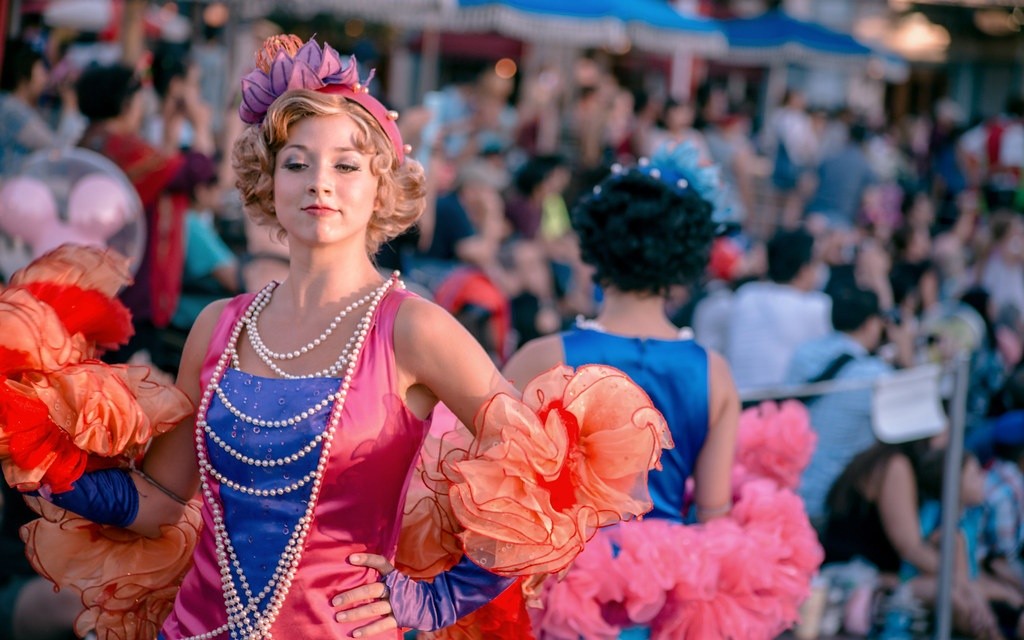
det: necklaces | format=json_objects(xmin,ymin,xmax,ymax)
[{"xmin": 185, "ymin": 277, "xmax": 394, "ymax": 640}]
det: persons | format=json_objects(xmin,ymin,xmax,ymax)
[
  {"xmin": 825, "ymin": 419, "xmax": 939, "ymax": 574},
  {"xmin": 501, "ymin": 159, "xmax": 741, "ymax": 639},
  {"xmin": 729, "ymin": 227, "xmax": 832, "ymax": 408},
  {"xmin": 142, "ymin": 44, "xmax": 198, "ymax": 147},
  {"xmin": 165, "ymin": 149, "xmax": 244, "ymax": 330},
  {"xmin": 18, "ymin": 35, "xmax": 575, "ymax": 640},
  {"xmin": 74, "ymin": 60, "xmax": 215, "ymax": 366},
  {"xmin": 788, "ymin": 288, "xmax": 896, "ymax": 527},
  {"xmin": 0, "ymin": 43, "xmax": 90, "ymax": 184},
  {"xmin": 391, "ymin": 31, "xmax": 1024, "ymax": 640},
  {"xmin": 0, "ymin": 0, "xmax": 279, "ymax": 193}
]
